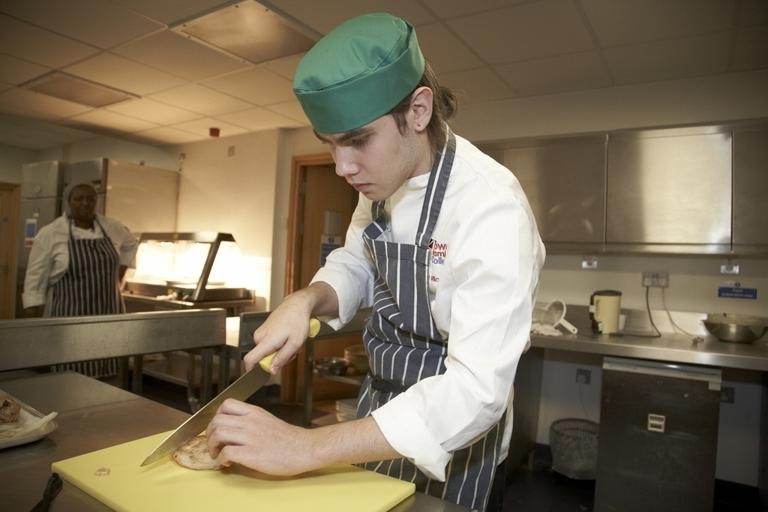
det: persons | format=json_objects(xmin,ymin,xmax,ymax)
[
  {"xmin": 204, "ymin": 11, "xmax": 545, "ymax": 511},
  {"xmin": 21, "ymin": 176, "xmax": 138, "ymax": 386}
]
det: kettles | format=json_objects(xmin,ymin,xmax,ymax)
[{"xmin": 588, "ymin": 289, "xmax": 623, "ymax": 335}]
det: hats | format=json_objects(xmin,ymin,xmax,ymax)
[
  {"xmin": 62, "ymin": 178, "xmax": 99, "ymax": 209},
  {"xmin": 292, "ymin": 11, "xmax": 427, "ymax": 136}
]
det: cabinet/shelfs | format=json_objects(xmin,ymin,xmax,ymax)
[{"xmin": 470, "ymin": 117, "xmax": 767, "ymax": 258}]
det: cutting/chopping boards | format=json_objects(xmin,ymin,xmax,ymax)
[{"xmin": 48, "ymin": 426, "xmax": 417, "ymax": 512}]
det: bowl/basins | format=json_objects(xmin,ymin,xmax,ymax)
[
  {"xmin": 701, "ymin": 317, "xmax": 767, "ymax": 344},
  {"xmin": 310, "ymin": 357, "xmax": 352, "ymax": 377}
]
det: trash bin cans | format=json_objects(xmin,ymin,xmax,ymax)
[{"xmin": 549, "ymin": 417, "xmax": 599, "ymax": 478}]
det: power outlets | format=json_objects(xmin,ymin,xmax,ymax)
[{"xmin": 642, "ymin": 271, "xmax": 670, "ymax": 288}]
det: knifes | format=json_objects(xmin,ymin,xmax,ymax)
[{"xmin": 136, "ymin": 317, "xmax": 323, "ymax": 467}]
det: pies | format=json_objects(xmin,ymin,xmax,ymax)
[{"xmin": 173, "ymin": 436, "xmax": 224, "ymax": 471}]
